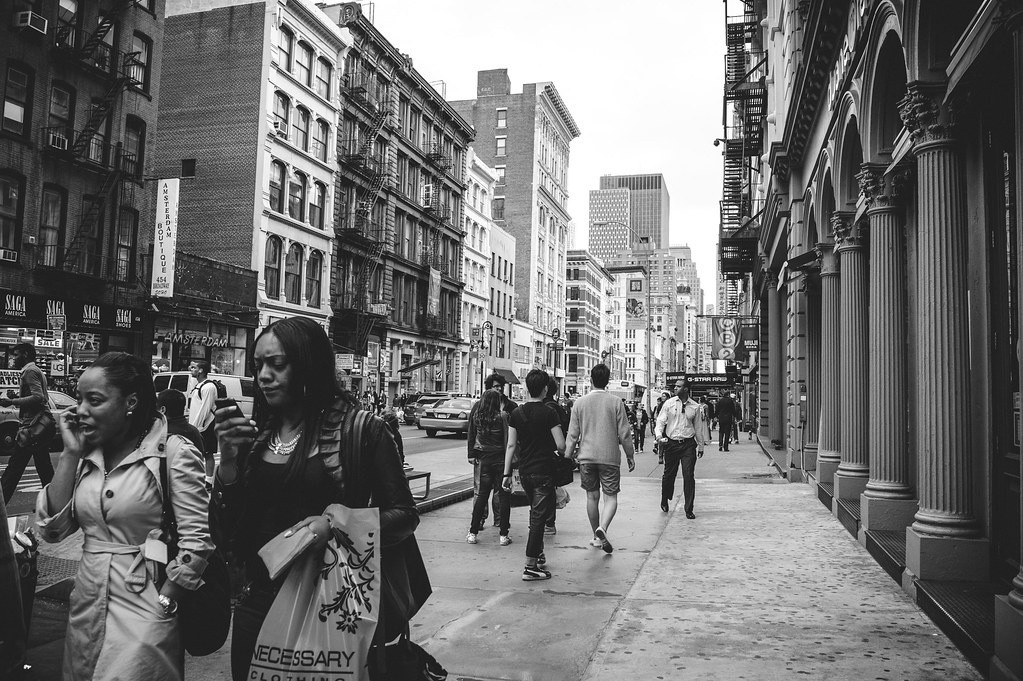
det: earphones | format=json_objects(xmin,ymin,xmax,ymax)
[{"xmin": 199, "ymin": 371, "xmax": 201, "ymax": 372}]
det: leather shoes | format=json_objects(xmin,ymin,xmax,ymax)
[
  {"xmin": 685, "ymin": 511, "xmax": 696, "ymax": 519},
  {"xmin": 660, "ymin": 499, "xmax": 669, "ymax": 513}
]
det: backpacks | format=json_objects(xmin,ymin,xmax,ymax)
[{"xmin": 196, "ymin": 379, "xmax": 229, "ymax": 416}]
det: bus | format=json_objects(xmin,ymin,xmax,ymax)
[{"xmin": 606, "ymin": 380, "xmax": 647, "ymax": 411}]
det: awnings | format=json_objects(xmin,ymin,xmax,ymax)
[{"xmin": 495, "ymin": 370, "xmax": 521, "ymax": 385}]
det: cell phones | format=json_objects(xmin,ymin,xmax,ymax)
[
  {"xmin": 214, "ymin": 398, "xmax": 245, "ymax": 419},
  {"xmin": 65, "ymin": 408, "xmax": 78, "ymax": 429}
]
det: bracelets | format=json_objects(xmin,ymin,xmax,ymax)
[
  {"xmin": 159, "ymin": 594, "xmax": 171, "ymax": 608},
  {"xmin": 502, "ymin": 474, "xmax": 513, "ymax": 477}
]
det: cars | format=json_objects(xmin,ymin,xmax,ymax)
[
  {"xmin": 0, "ymin": 390, "xmax": 80, "ymax": 455},
  {"xmin": 404, "ymin": 391, "xmax": 481, "ymax": 438}
]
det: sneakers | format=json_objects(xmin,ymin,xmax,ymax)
[
  {"xmin": 538, "ymin": 553, "xmax": 547, "ymax": 563},
  {"xmin": 523, "ymin": 565, "xmax": 551, "ymax": 580}
]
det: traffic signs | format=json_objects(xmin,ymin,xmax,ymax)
[{"xmin": 546, "ymin": 342, "xmax": 563, "ymax": 348}]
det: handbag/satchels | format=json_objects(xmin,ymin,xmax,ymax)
[
  {"xmin": 164, "ymin": 534, "xmax": 240, "ymax": 659},
  {"xmin": 552, "ymin": 456, "xmax": 576, "ymax": 486},
  {"xmin": 511, "ymin": 467, "xmax": 528, "ymax": 495},
  {"xmin": 15, "ymin": 409, "xmax": 68, "ymax": 454},
  {"xmin": 350, "ymin": 410, "xmax": 438, "ymax": 632},
  {"xmin": 258, "ymin": 520, "xmax": 319, "ymax": 578},
  {"xmin": 240, "ymin": 502, "xmax": 383, "ymax": 681}
]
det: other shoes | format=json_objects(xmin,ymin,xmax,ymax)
[
  {"xmin": 500, "ymin": 535, "xmax": 512, "ymax": 545},
  {"xmin": 719, "ymin": 447, "xmax": 723, "ymax": 451},
  {"xmin": 464, "ymin": 530, "xmax": 480, "ymax": 543},
  {"xmin": 652, "ymin": 448, "xmax": 659, "ymax": 454},
  {"xmin": 595, "ymin": 527, "xmax": 613, "ymax": 553},
  {"xmin": 494, "ymin": 523, "xmax": 502, "ymax": 527},
  {"xmin": 640, "ymin": 450, "xmax": 644, "ymax": 453},
  {"xmin": 479, "ymin": 521, "xmax": 484, "ymax": 529},
  {"xmin": 589, "ymin": 538, "xmax": 604, "ymax": 548},
  {"xmin": 541, "ymin": 525, "xmax": 557, "ymax": 535},
  {"xmin": 724, "ymin": 450, "xmax": 729, "ymax": 452},
  {"xmin": 634, "ymin": 449, "xmax": 638, "ymax": 454}
]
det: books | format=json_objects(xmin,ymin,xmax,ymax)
[{"xmin": 257, "ymin": 520, "xmax": 318, "ymax": 580}]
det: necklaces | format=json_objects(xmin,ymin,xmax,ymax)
[
  {"xmin": 133, "ymin": 428, "xmax": 148, "ymax": 451},
  {"xmin": 266, "ymin": 406, "xmax": 326, "ymax": 456}
]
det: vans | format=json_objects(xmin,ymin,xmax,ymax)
[{"xmin": 152, "ymin": 372, "xmax": 254, "ymax": 420}]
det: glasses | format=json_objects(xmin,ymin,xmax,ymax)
[
  {"xmin": 491, "ymin": 384, "xmax": 507, "ymax": 389},
  {"xmin": 681, "ymin": 403, "xmax": 687, "ymax": 414}
]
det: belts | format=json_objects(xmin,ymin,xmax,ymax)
[{"xmin": 669, "ymin": 438, "xmax": 692, "ymax": 443}]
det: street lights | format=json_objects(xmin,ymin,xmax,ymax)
[
  {"xmin": 481, "ymin": 321, "xmax": 495, "ymax": 397},
  {"xmin": 594, "ymin": 221, "xmax": 651, "ymax": 419}
]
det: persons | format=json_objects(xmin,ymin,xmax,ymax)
[
  {"xmin": 188, "ymin": 360, "xmax": 220, "ymax": 493},
  {"xmin": 467, "ymin": 374, "xmax": 518, "ymax": 531},
  {"xmin": 393, "ymin": 392, "xmax": 410, "ymax": 423},
  {"xmin": 621, "ymin": 392, "xmax": 742, "ymax": 464},
  {"xmin": 564, "ymin": 364, "xmax": 637, "ymax": 553},
  {"xmin": 34, "ymin": 348, "xmax": 217, "ymax": 681},
  {"xmin": 464, "ymin": 389, "xmax": 513, "ymax": 546},
  {"xmin": 501, "ymin": 368, "xmax": 573, "ymax": 582},
  {"xmin": 209, "ymin": 316, "xmax": 435, "ymax": 681},
  {"xmin": 0, "ymin": 343, "xmax": 55, "ymax": 506},
  {"xmin": 655, "ymin": 377, "xmax": 704, "ymax": 519},
  {"xmin": 353, "ymin": 390, "xmax": 387, "ymax": 415},
  {"xmin": 152, "ymin": 363, "xmax": 169, "ymax": 373},
  {"xmin": 156, "ymin": 389, "xmax": 206, "ymax": 457}
]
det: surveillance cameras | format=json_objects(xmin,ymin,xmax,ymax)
[{"xmin": 714, "ymin": 141, "xmax": 719, "ymax": 146}]
